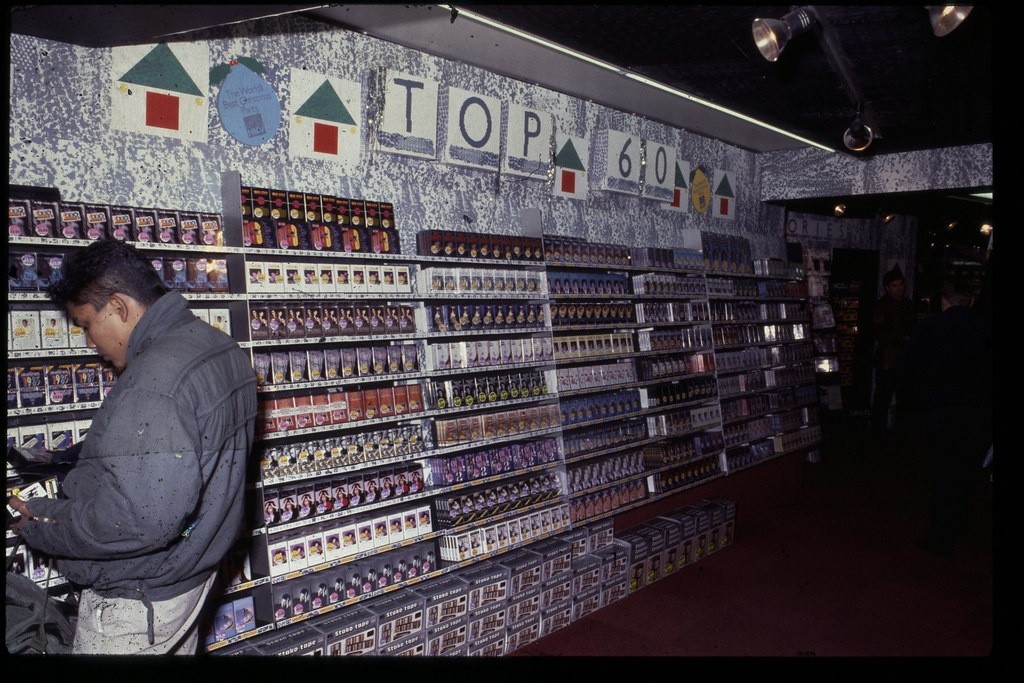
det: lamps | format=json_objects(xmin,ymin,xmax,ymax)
[
  {"xmin": 752, "ymin": 4, "xmax": 884, "ymax": 152},
  {"xmin": 923, "ymin": 5, "xmax": 973, "ymax": 38}
]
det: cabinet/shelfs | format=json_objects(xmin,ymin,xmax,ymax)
[{"xmin": 9, "ymin": 170, "xmax": 827, "ymax": 654}]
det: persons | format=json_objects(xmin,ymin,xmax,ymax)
[
  {"xmin": 895, "ymin": 260, "xmax": 990, "ymax": 550},
  {"xmin": 4, "ymin": 238, "xmax": 258, "ymax": 654}
]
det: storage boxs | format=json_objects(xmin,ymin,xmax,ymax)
[{"xmin": 9, "ymin": 184, "xmax": 845, "ymax": 655}]
